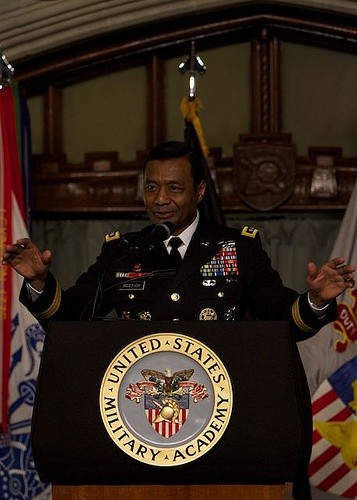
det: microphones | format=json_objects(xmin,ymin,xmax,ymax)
[
  {"xmin": 142, "ymin": 221, "xmax": 174, "ymax": 251},
  {"xmin": 79, "ymin": 269, "xmax": 177, "ymax": 322}
]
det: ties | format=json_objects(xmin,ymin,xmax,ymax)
[{"xmin": 168, "ymin": 237, "xmax": 184, "ymax": 264}]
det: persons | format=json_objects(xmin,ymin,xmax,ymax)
[{"xmin": 2, "ymin": 140, "xmax": 357, "ymax": 345}]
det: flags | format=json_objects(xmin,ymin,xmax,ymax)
[
  {"xmin": 0, "ymin": 80, "xmax": 50, "ymax": 500},
  {"xmin": 180, "ymin": 99, "xmax": 228, "ymax": 227},
  {"xmin": 296, "ymin": 174, "xmax": 357, "ymax": 500}
]
